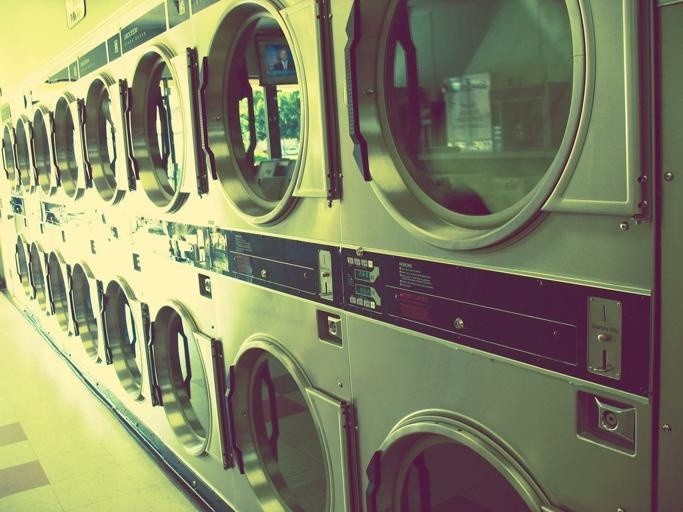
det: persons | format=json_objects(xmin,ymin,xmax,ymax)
[{"xmin": 273, "ymin": 49, "xmax": 295, "ymax": 71}]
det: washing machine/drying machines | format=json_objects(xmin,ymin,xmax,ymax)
[{"xmin": 0, "ymin": 0, "xmax": 683, "ymax": 511}]
